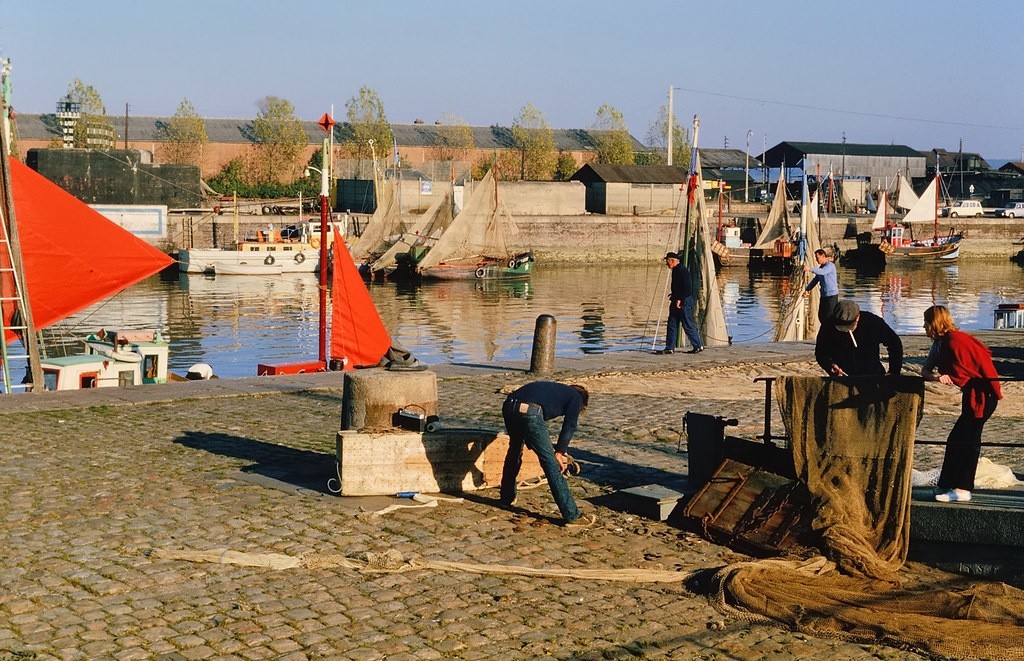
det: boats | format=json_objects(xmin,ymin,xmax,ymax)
[
  {"xmin": 178, "ymin": 191, "xmax": 348, "ymax": 275},
  {"xmin": 200, "ymin": 180, "xmax": 317, "ymax": 215}
]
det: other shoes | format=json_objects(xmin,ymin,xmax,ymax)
[
  {"xmin": 656, "ymin": 349, "xmax": 674, "ymax": 354},
  {"xmin": 688, "ymin": 346, "xmax": 705, "ymax": 354}
]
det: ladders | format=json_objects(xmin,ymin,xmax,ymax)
[
  {"xmin": 0, "ymin": 208, "xmax": 34, "ymax": 395},
  {"xmin": 822, "ymin": 183, "xmax": 829, "ymax": 212},
  {"xmin": 832, "ymin": 181, "xmax": 842, "ymax": 213}
]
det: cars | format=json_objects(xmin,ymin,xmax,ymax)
[
  {"xmin": 942, "ymin": 200, "xmax": 984, "ymax": 218},
  {"xmin": 994, "ymin": 202, "xmax": 1024, "ymax": 218}
]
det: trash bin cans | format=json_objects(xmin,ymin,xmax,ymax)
[
  {"xmin": 946, "ymin": 198, "xmax": 953, "ymax": 208},
  {"xmin": 634, "ymin": 206, "xmax": 638, "ymax": 216},
  {"xmin": 686, "ymin": 412, "xmax": 726, "ymax": 476}
]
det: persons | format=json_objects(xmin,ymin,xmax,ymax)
[
  {"xmin": 802, "ymin": 249, "xmax": 839, "ymax": 323},
  {"xmin": 814, "ymin": 299, "xmax": 903, "ymax": 376},
  {"xmin": 921, "ymin": 306, "xmax": 1003, "ymax": 502},
  {"xmin": 500, "ymin": 380, "xmax": 595, "ymax": 527},
  {"xmin": 656, "ymin": 252, "xmax": 704, "ymax": 354}
]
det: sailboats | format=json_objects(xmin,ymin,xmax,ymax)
[
  {"xmin": 350, "ymin": 140, "xmax": 537, "ymax": 279},
  {"xmin": 0, "ymin": 56, "xmax": 421, "ymax": 392},
  {"xmin": 636, "ymin": 116, "xmax": 822, "ymax": 349},
  {"xmin": 871, "ymin": 165, "xmax": 964, "ymax": 263}
]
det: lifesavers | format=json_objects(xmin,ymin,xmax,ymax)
[
  {"xmin": 310, "ymin": 237, "xmax": 321, "ymax": 249},
  {"xmin": 508, "ymin": 260, "xmax": 515, "ymax": 267},
  {"xmin": 474, "ymin": 267, "xmax": 485, "ymax": 277},
  {"xmin": 272, "ymin": 206, "xmax": 280, "ymax": 215},
  {"xmin": 262, "ymin": 205, "xmax": 271, "ymax": 215},
  {"xmin": 265, "ymin": 255, "xmax": 275, "ymax": 266},
  {"xmin": 295, "ymin": 252, "xmax": 304, "ymax": 263}
]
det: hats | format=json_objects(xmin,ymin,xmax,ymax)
[
  {"xmin": 664, "ymin": 252, "xmax": 681, "ymax": 259},
  {"xmin": 833, "ymin": 300, "xmax": 860, "ymax": 332}
]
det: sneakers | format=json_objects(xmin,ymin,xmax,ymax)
[
  {"xmin": 502, "ymin": 497, "xmax": 518, "ymax": 507},
  {"xmin": 935, "ymin": 489, "xmax": 971, "ymax": 502},
  {"xmin": 933, "ymin": 486, "xmax": 954, "ymax": 494},
  {"xmin": 565, "ymin": 513, "xmax": 596, "ymax": 527}
]
